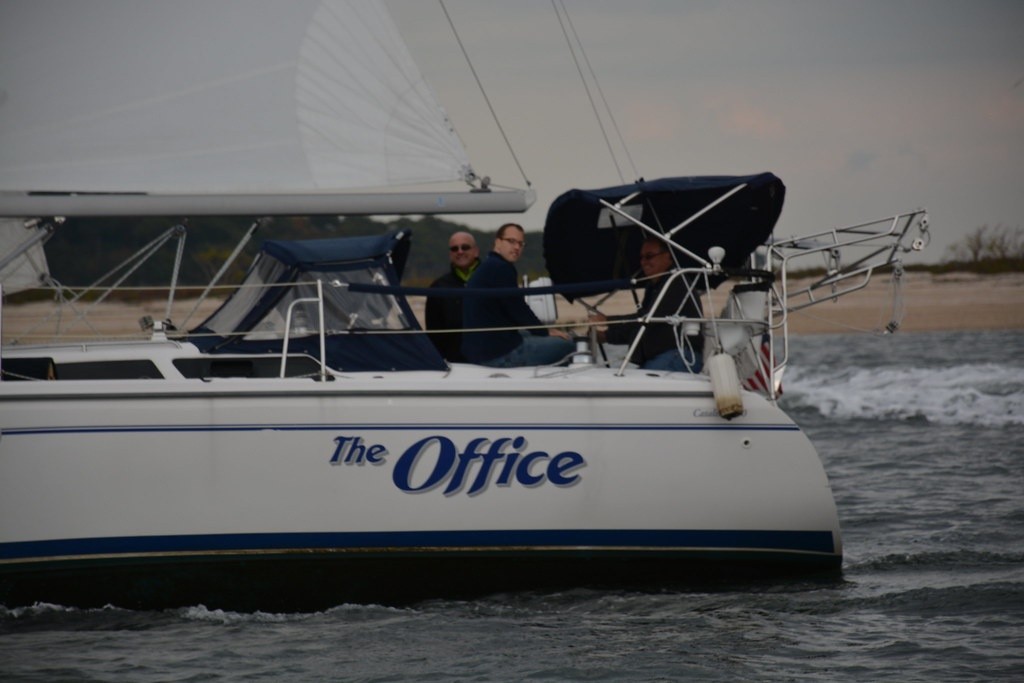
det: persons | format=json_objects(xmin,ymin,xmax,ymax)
[
  {"xmin": 581, "ymin": 236, "xmax": 705, "ymax": 373},
  {"xmin": 425, "ymin": 223, "xmax": 577, "ymax": 367}
]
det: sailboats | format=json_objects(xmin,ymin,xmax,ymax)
[{"xmin": 0, "ymin": 2, "xmax": 934, "ymax": 616}]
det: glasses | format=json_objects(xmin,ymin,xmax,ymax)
[
  {"xmin": 638, "ymin": 252, "xmax": 665, "ymax": 261},
  {"xmin": 449, "ymin": 243, "xmax": 477, "ymax": 252},
  {"xmin": 499, "ymin": 236, "xmax": 525, "ymax": 249}
]
